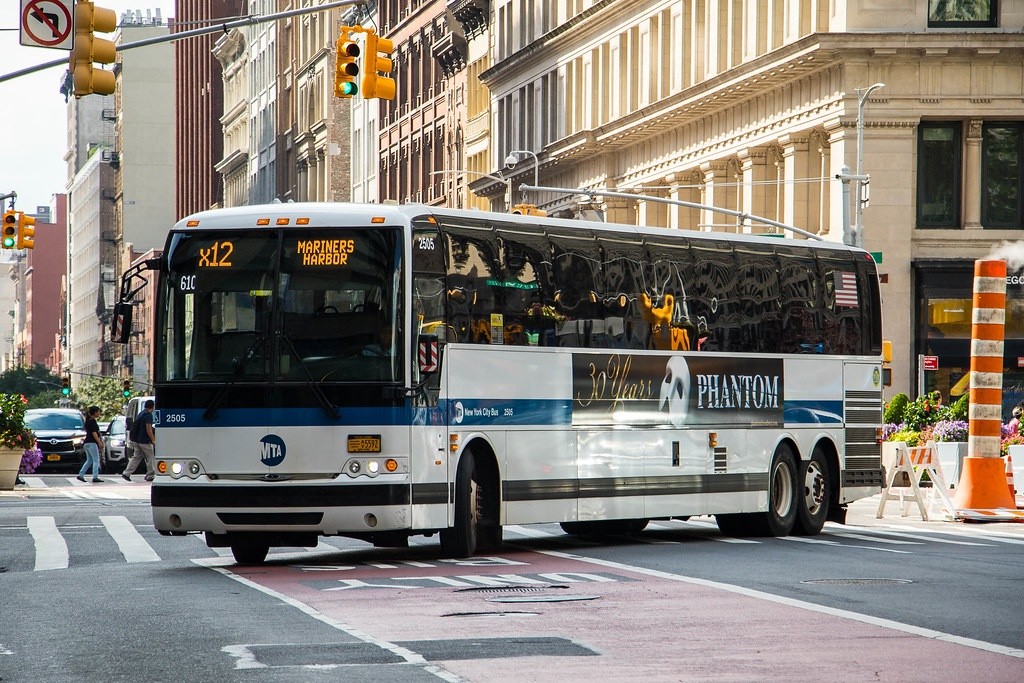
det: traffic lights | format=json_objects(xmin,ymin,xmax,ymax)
[
  {"xmin": 361, "ymin": 32, "xmax": 397, "ymax": 101},
  {"xmin": 509, "ymin": 205, "xmax": 548, "ymax": 217},
  {"xmin": 68, "ymin": 0, "xmax": 117, "ymax": 98},
  {"xmin": 1, "ymin": 210, "xmax": 17, "ymax": 249},
  {"xmin": 122, "ymin": 380, "xmax": 130, "ymax": 397},
  {"xmin": 17, "ymin": 212, "xmax": 37, "ymax": 250},
  {"xmin": 335, "ymin": 39, "xmax": 359, "ymax": 101},
  {"xmin": 62, "ymin": 376, "xmax": 70, "ymax": 394}
]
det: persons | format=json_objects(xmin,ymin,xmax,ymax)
[
  {"xmin": 930, "ymin": 391, "xmax": 949, "ymax": 415},
  {"xmin": 122, "ymin": 400, "xmax": 155, "ymax": 481},
  {"xmin": 76, "ymin": 406, "xmax": 105, "ymax": 482},
  {"xmin": 1009, "ymin": 406, "xmax": 1023, "ymax": 434}
]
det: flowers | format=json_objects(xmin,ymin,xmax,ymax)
[
  {"xmin": 932, "ymin": 420, "xmax": 969, "ymax": 442},
  {"xmin": 1001, "ymin": 424, "xmax": 1011, "ymax": 442},
  {"xmin": 18, "ymin": 442, "xmax": 42, "ymax": 474},
  {"xmin": 882, "ymin": 423, "xmax": 903, "ymax": 441}
]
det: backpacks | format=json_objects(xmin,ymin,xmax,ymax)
[{"xmin": 130, "ymin": 412, "xmax": 151, "ymax": 441}]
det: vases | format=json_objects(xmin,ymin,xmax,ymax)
[
  {"xmin": 882, "ymin": 443, "xmax": 904, "ymax": 485},
  {"xmin": 935, "ymin": 443, "xmax": 969, "ymax": 491},
  {"xmin": 1010, "ymin": 445, "xmax": 1024, "ymax": 508}
]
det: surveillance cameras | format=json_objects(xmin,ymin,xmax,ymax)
[{"xmin": 505, "ymin": 156, "xmax": 518, "ymax": 169}]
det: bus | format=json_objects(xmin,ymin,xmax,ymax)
[{"xmin": 107, "ymin": 200, "xmax": 890, "ymax": 564}]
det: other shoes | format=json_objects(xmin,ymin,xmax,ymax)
[
  {"xmin": 93, "ymin": 478, "xmax": 104, "ymax": 482},
  {"xmin": 122, "ymin": 474, "xmax": 131, "ymax": 481},
  {"xmin": 77, "ymin": 476, "xmax": 87, "ymax": 482},
  {"xmin": 145, "ymin": 476, "xmax": 153, "ymax": 481}
]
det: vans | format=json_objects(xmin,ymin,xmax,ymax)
[{"xmin": 124, "ymin": 395, "xmax": 155, "ymax": 473}]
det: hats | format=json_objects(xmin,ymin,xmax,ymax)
[{"xmin": 1012, "ymin": 406, "xmax": 1022, "ymax": 416}]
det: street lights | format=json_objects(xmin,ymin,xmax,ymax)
[{"xmin": 852, "ymin": 82, "xmax": 886, "ymax": 249}]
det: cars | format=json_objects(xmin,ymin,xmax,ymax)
[
  {"xmin": 100, "ymin": 416, "xmax": 128, "ymax": 472},
  {"xmin": 19, "ymin": 405, "xmax": 90, "ymax": 472},
  {"xmin": 98, "ymin": 421, "xmax": 111, "ymax": 435}
]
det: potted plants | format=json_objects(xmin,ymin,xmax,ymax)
[{"xmin": 0, "ymin": 393, "xmax": 36, "ymax": 491}]
